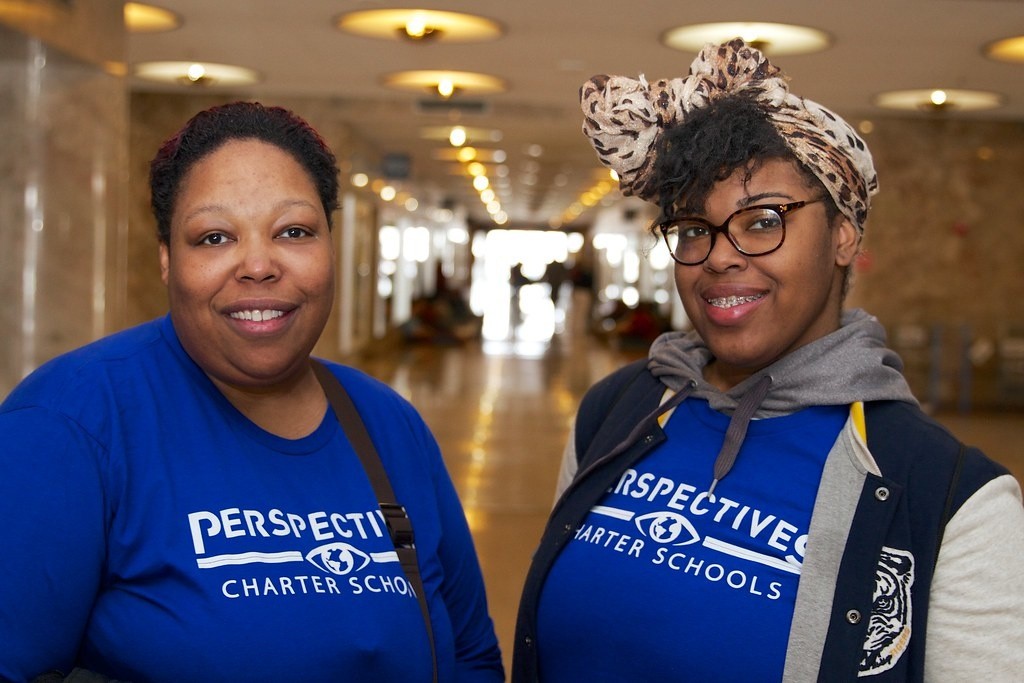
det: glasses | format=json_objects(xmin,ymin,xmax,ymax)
[{"xmin": 659, "ymin": 198, "xmax": 830, "ymax": 266}]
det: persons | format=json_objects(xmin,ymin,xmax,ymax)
[
  {"xmin": 512, "ymin": 38, "xmax": 1024, "ymax": 683},
  {"xmin": 596, "ymin": 299, "xmax": 666, "ymax": 352},
  {"xmin": 417, "ymin": 258, "xmax": 474, "ymax": 347},
  {"xmin": 509, "ymin": 261, "xmax": 595, "ymax": 304},
  {"xmin": 0, "ymin": 101, "xmax": 506, "ymax": 683}
]
